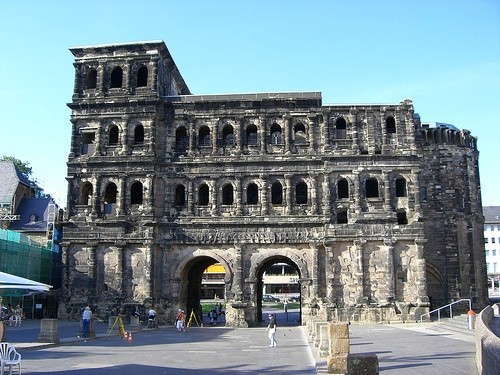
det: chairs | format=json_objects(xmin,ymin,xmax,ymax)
[{"xmin": 0, "ymin": 342, "xmax": 22, "ymax": 375}]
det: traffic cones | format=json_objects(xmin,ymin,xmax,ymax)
[
  {"xmin": 122, "ymin": 331, "xmax": 128, "ymax": 341},
  {"xmin": 127, "ymin": 332, "xmax": 134, "ymax": 342}
]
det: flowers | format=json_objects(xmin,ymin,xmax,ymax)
[{"xmin": 0, "ymin": 306, "xmax": 12, "ymax": 321}]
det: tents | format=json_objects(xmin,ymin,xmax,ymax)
[{"xmin": 0, "ymin": 271, "xmax": 54, "ymax": 319}]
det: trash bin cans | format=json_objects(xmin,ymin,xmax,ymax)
[{"xmin": 468, "ymin": 310, "xmax": 476, "ymax": 330}]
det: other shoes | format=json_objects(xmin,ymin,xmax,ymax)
[
  {"xmin": 274, "ymin": 343, "xmax": 276, "ymax": 346},
  {"xmin": 270, "ymin": 345, "xmax": 273, "ymax": 347}
]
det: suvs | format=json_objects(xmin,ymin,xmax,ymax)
[{"xmin": 261, "ymin": 295, "xmax": 280, "ymax": 303}]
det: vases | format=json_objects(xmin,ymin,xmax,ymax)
[{"xmin": 0, "ymin": 322, "xmax": 5, "ymax": 342}]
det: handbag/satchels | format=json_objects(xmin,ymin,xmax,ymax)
[{"xmin": 176, "ymin": 319, "xmax": 178, "ymax": 321}]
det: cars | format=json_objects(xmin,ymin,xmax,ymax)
[{"xmin": 288, "ymin": 295, "xmax": 305, "ymax": 303}]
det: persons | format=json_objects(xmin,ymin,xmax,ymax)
[
  {"xmin": 132, "ymin": 305, "xmax": 141, "ymax": 320},
  {"xmin": 176, "ymin": 309, "xmax": 186, "ymax": 332},
  {"xmin": 147, "ymin": 307, "xmax": 157, "ymax": 318},
  {"xmin": 283, "ymin": 299, "xmax": 288, "ymax": 312},
  {"xmin": 265, "ymin": 314, "xmax": 277, "ymax": 347},
  {"xmin": 8, "ymin": 305, "xmax": 14, "ymax": 326},
  {"xmin": 208, "ymin": 303, "xmax": 222, "ymax": 323},
  {"xmin": 81, "ymin": 306, "xmax": 92, "ymax": 338},
  {"xmin": 14, "ymin": 304, "xmax": 23, "ymax": 327}
]
